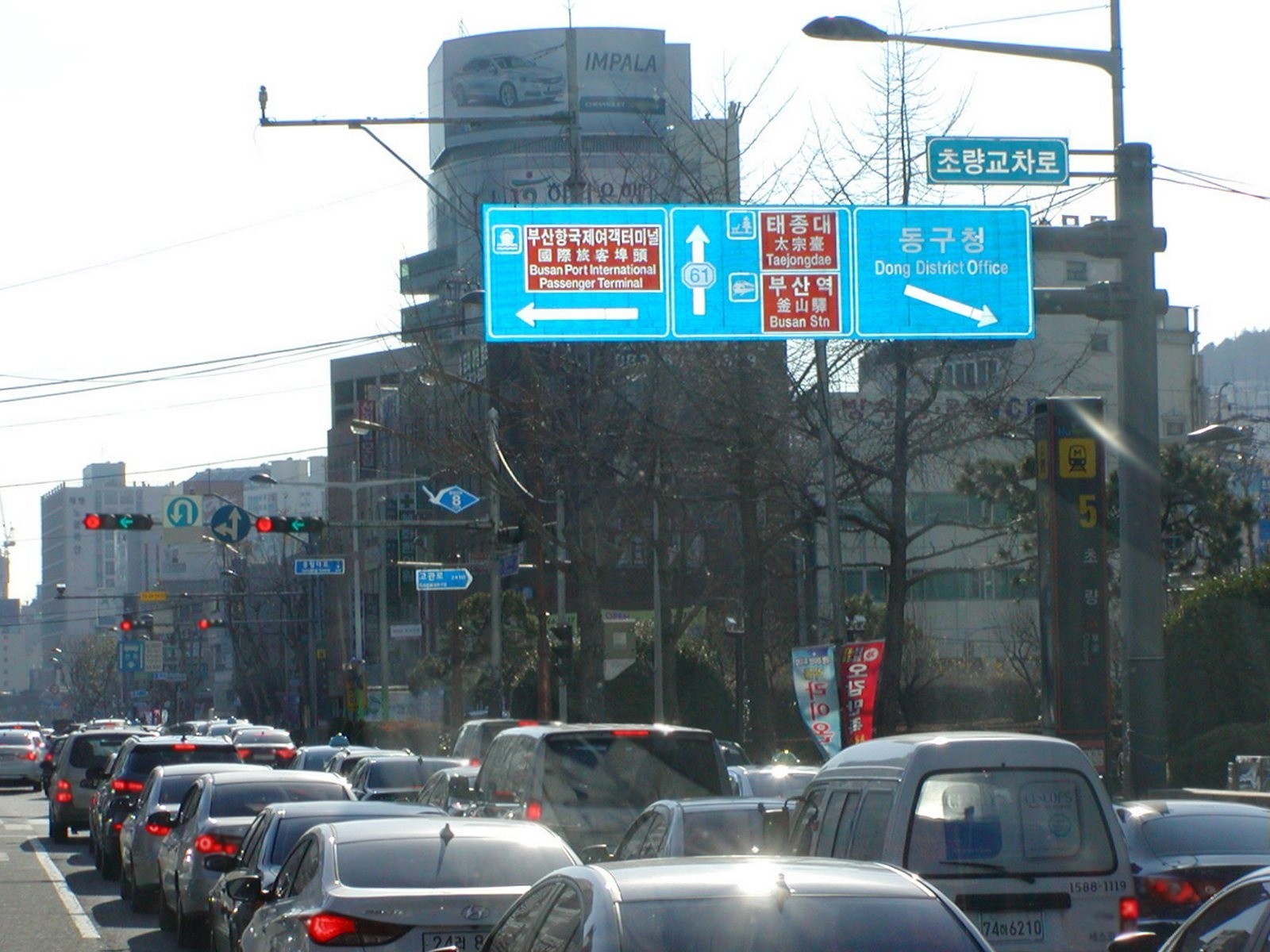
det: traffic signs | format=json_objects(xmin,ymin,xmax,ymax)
[
  {"xmin": 415, "ymin": 568, "xmax": 474, "ymax": 591},
  {"xmin": 854, "ymin": 204, "xmax": 1035, "ymax": 341},
  {"xmin": 671, "ymin": 205, "xmax": 854, "ymax": 342},
  {"xmin": 481, "ymin": 201, "xmax": 670, "ymax": 344}
]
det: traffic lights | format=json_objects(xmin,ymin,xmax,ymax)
[
  {"xmin": 196, "ymin": 618, "xmax": 225, "ymax": 629},
  {"xmin": 256, "ymin": 516, "xmax": 326, "ymax": 534},
  {"xmin": 82, "ymin": 512, "xmax": 154, "ymax": 531},
  {"xmin": 119, "ymin": 620, "xmax": 152, "ymax": 632}
]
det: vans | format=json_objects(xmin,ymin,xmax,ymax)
[
  {"xmin": 447, "ymin": 721, "xmax": 735, "ymax": 864},
  {"xmin": 761, "ymin": 729, "xmax": 1139, "ymax": 952}
]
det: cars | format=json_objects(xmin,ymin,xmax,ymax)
[
  {"xmin": 725, "ymin": 764, "xmax": 822, "ymax": 799},
  {"xmin": 716, "ymin": 739, "xmax": 754, "ymax": 767},
  {"xmin": 450, "ymin": 52, "xmax": 568, "ymax": 110},
  {"xmin": 1106, "ymin": 865, "xmax": 1270, "ymax": 952},
  {"xmin": 202, "ymin": 800, "xmax": 458, "ymax": 952},
  {"xmin": 1112, "ymin": 797, "xmax": 1270, "ymax": 949},
  {"xmin": 224, "ymin": 814, "xmax": 587, "ymax": 952},
  {"xmin": 424, "ymin": 852, "xmax": 996, "ymax": 952},
  {"xmin": 0, "ymin": 716, "xmax": 483, "ymax": 950},
  {"xmin": 578, "ymin": 794, "xmax": 801, "ymax": 864}
]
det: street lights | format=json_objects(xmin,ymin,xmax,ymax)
[{"xmin": 801, "ymin": 0, "xmax": 1173, "ymax": 797}]
